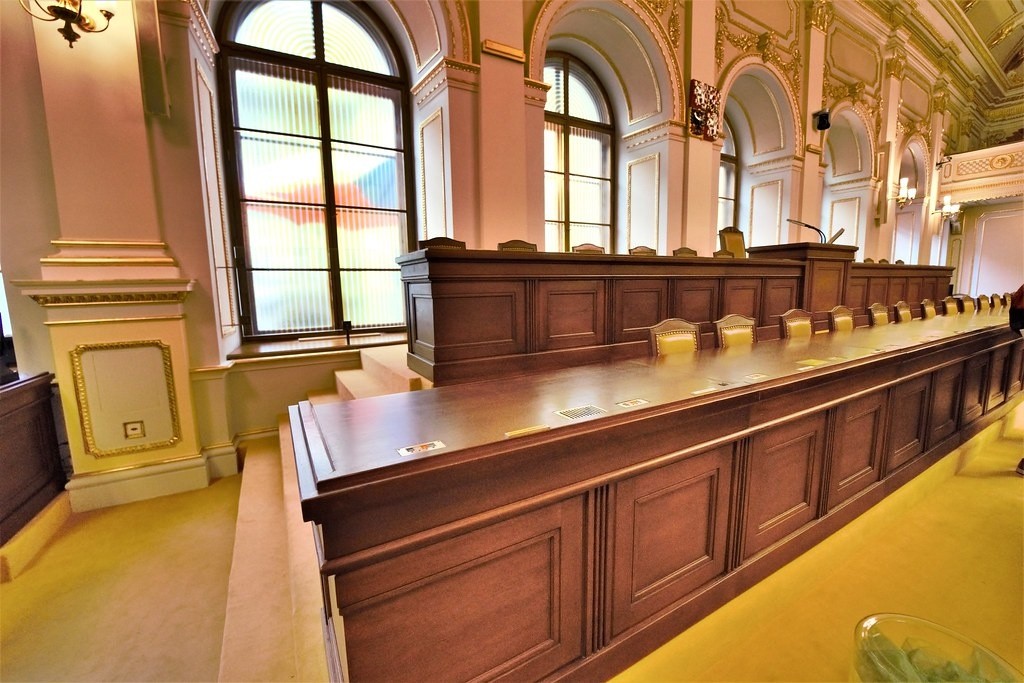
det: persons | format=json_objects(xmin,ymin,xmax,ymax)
[{"xmin": 1009, "ymin": 283, "xmax": 1024, "ymax": 475}]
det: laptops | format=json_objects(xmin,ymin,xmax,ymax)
[{"xmin": 827, "ymin": 229, "xmax": 845, "ymax": 244}]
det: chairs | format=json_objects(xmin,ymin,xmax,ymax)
[
  {"xmin": 940, "ymin": 296, "xmax": 958, "ymax": 315},
  {"xmin": 867, "ymin": 302, "xmax": 890, "ymax": 327},
  {"xmin": 628, "ymin": 245, "xmax": 657, "ymax": 255},
  {"xmin": 893, "ymin": 301, "xmax": 912, "ymax": 323},
  {"xmin": 864, "ymin": 258, "xmax": 874, "ymax": 263},
  {"xmin": 416, "ymin": 237, "xmax": 467, "ymax": 250},
  {"xmin": 977, "ymin": 294, "xmax": 990, "ymax": 311},
  {"xmin": 673, "ymin": 247, "xmax": 698, "ymax": 256},
  {"xmin": 896, "ymin": 260, "xmax": 904, "ymax": 264},
  {"xmin": 828, "ymin": 305, "xmax": 856, "ymax": 331},
  {"xmin": 713, "ymin": 313, "xmax": 758, "ymax": 348},
  {"xmin": 1003, "ymin": 293, "xmax": 1011, "ymax": 306},
  {"xmin": 780, "ymin": 308, "xmax": 816, "ymax": 340},
  {"xmin": 719, "ymin": 227, "xmax": 746, "ymax": 258},
  {"xmin": 990, "ymin": 293, "xmax": 1002, "ymax": 309},
  {"xmin": 572, "ymin": 243, "xmax": 605, "ymax": 254},
  {"xmin": 959, "ymin": 295, "xmax": 975, "ymax": 312},
  {"xmin": 497, "ymin": 240, "xmax": 537, "ymax": 252},
  {"xmin": 920, "ymin": 299, "xmax": 937, "ymax": 319},
  {"xmin": 879, "ymin": 259, "xmax": 889, "ymax": 264},
  {"xmin": 649, "ymin": 317, "xmax": 702, "ymax": 356},
  {"xmin": 713, "ymin": 249, "xmax": 734, "ymax": 258}
]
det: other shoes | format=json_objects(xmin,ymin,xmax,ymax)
[{"xmin": 1016, "ymin": 458, "xmax": 1024, "ymax": 475}]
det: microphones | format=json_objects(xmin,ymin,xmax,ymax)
[{"xmin": 787, "ymin": 219, "xmax": 826, "ymax": 242}]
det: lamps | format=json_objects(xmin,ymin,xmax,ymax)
[
  {"xmin": 19, "ymin": 0, "xmax": 115, "ymax": 48},
  {"xmin": 931, "ymin": 195, "xmax": 963, "ymax": 220},
  {"xmin": 892, "ymin": 177, "xmax": 917, "ymax": 209},
  {"xmin": 813, "ymin": 107, "xmax": 832, "ymax": 130}
]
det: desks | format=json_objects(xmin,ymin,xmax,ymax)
[
  {"xmin": 283, "ymin": 310, "xmax": 1024, "ymax": 683},
  {"xmin": 848, "ymin": 262, "xmax": 956, "ymax": 327},
  {"xmin": 394, "ymin": 247, "xmax": 807, "ymax": 384}
]
